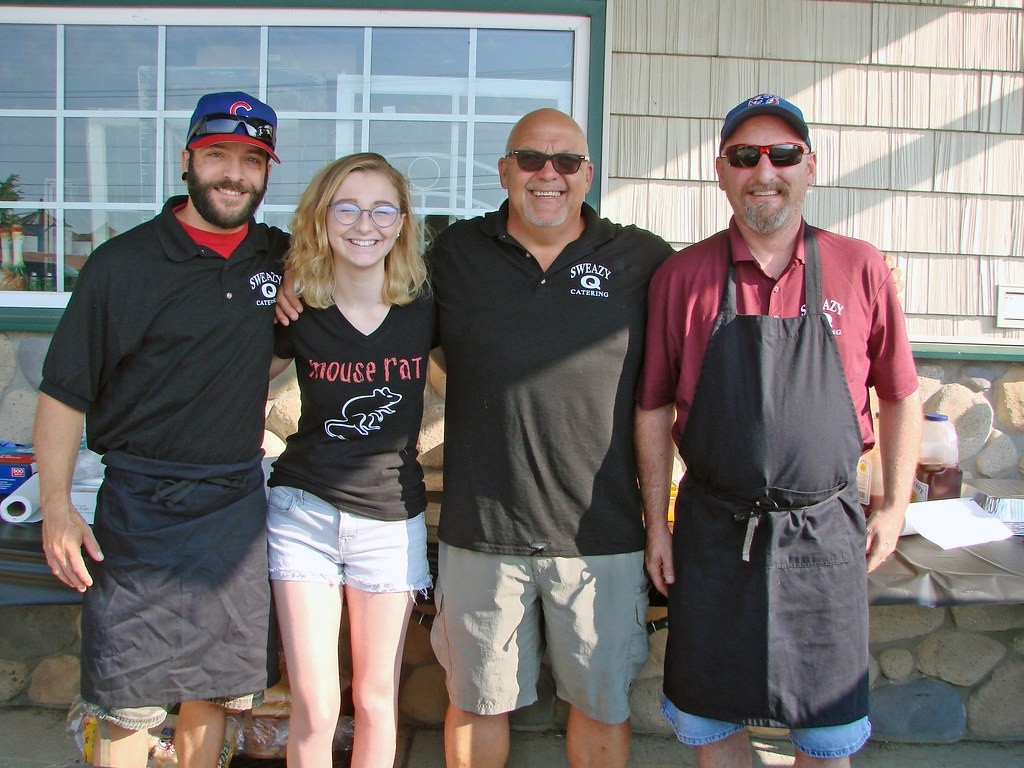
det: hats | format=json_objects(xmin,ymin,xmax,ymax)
[
  {"xmin": 720, "ymin": 94, "xmax": 812, "ymax": 153},
  {"xmin": 183, "ymin": 91, "xmax": 280, "ymax": 164}
]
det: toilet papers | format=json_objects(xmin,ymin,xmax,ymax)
[
  {"xmin": 899, "ymin": 498, "xmax": 1013, "ymax": 551},
  {"xmin": 0, "ymin": 473, "xmax": 41, "ymax": 525}
]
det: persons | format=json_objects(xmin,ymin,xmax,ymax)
[
  {"xmin": 269, "ymin": 153, "xmax": 447, "ymax": 768},
  {"xmin": 34, "ymin": 90, "xmax": 292, "ymax": 768},
  {"xmin": 274, "ymin": 108, "xmax": 904, "ymax": 768},
  {"xmin": 635, "ymin": 93, "xmax": 923, "ymax": 768}
]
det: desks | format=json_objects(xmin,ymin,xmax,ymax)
[{"xmin": 0, "ymin": 519, "xmax": 1024, "ymax": 603}]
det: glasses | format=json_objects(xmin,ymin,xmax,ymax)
[
  {"xmin": 327, "ymin": 201, "xmax": 404, "ymax": 228},
  {"xmin": 504, "ymin": 149, "xmax": 589, "ymax": 174},
  {"xmin": 720, "ymin": 140, "xmax": 809, "ymax": 167},
  {"xmin": 184, "ymin": 113, "xmax": 276, "ymax": 153}
]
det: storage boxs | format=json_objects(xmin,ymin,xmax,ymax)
[{"xmin": 0, "ymin": 453, "xmax": 39, "ymax": 495}]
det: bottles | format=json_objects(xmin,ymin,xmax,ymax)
[
  {"xmin": 856, "ymin": 412, "xmax": 886, "ymax": 517},
  {"xmin": 29, "ymin": 272, "xmax": 41, "ymax": 291},
  {"xmin": 42, "ymin": 272, "xmax": 54, "ymax": 291},
  {"xmin": 911, "ymin": 412, "xmax": 962, "ymax": 501}
]
